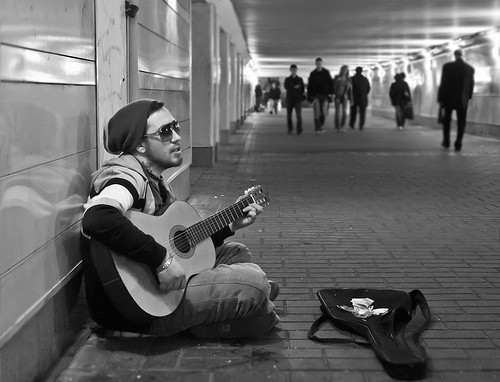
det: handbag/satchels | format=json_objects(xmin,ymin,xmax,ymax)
[{"xmin": 334, "ymin": 94, "xmax": 344, "ymax": 103}]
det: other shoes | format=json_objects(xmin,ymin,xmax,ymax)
[
  {"xmin": 397, "ymin": 125, "xmax": 404, "ymax": 130},
  {"xmin": 315, "ymin": 129, "xmax": 325, "ymax": 134},
  {"xmin": 190, "ymin": 279, "xmax": 281, "ymax": 339},
  {"xmin": 336, "ymin": 127, "xmax": 345, "ymax": 132}
]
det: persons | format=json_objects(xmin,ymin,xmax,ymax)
[
  {"xmin": 284, "ymin": 64, "xmax": 305, "ymax": 135},
  {"xmin": 307, "ymin": 57, "xmax": 371, "ymax": 135},
  {"xmin": 436, "ymin": 49, "xmax": 475, "ymax": 151},
  {"xmin": 389, "ymin": 72, "xmax": 413, "ymax": 130},
  {"xmin": 79, "ymin": 99, "xmax": 279, "ymax": 339},
  {"xmin": 255, "ymin": 76, "xmax": 281, "ymax": 116}
]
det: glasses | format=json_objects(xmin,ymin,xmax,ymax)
[{"xmin": 141, "ymin": 120, "xmax": 181, "ymax": 142}]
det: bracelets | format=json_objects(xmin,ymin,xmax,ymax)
[{"xmin": 156, "ymin": 253, "xmax": 174, "ymax": 273}]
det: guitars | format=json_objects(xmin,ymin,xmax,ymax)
[{"xmin": 91, "ymin": 184, "xmax": 269, "ymax": 333}]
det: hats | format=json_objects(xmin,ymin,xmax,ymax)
[{"xmin": 102, "ymin": 98, "xmax": 157, "ymax": 155}]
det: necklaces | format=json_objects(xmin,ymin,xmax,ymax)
[{"xmin": 144, "ymin": 167, "xmax": 163, "ymax": 182}]
